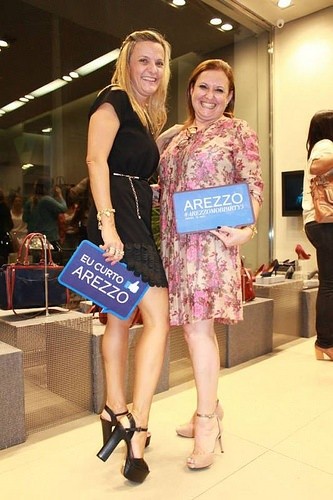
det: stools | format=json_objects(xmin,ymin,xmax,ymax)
[
  {"xmin": 43, "ymin": 317, "xmax": 173, "ymax": 413},
  {"xmin": 216, "ymin": 297, "xmax": 274, "ymax": 370},
  {"xmin": 303, "ymin": 286, "xmax": 319, "ymax": 338},
  {"xmin": 0, "ymin": 339, "xmax": 28, "ymax": 450},
  {"xmin": 0, "ymin": 306, "xmax": 87, "ymax": 369}
]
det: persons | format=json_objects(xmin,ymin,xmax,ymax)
[
  {"xmin": 86, "ymin": 30, "xmax": 183, "ymax": 484},
  {"xmin": 303, "ymin": 110, "xmax": 333, "ymax": 361},
  {"xmin": 159, "ymin": 59, "xmax": 264, "ymax": 469},
  {"xmin": 0, "ymin": 175, "xmax": 105, "ymax": 266}
]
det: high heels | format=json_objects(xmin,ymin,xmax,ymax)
[
  {"xmin": 251, "ymin": 264, "xmax": 268, "ymax": 282},
  {"xmin": 175, "ymin": 399, "xmax": 224, "ymax": 438},
  {"xmin": 260, "ymin": 258, "xmax": 279, "ymax": 277},
  {"xmin": 187, "ymin": 408, "xmax": 223, "ymax": 470},
  {"xmin": 277, "ymin": 259, "xmax": 296, "ymax": 278},
  {"xmin": 100, "ymin": 404, "xmax": 150, "ymax": 448},
  {"xmin": 315, "ymin": 341, "xmax": 333, "ymax": 360},
  {"xmin": 97, "ymin": 413, "xmax": 150, "ymax": 484},
  {"xmin": 295, "ymin": 244, "xmax": 310, "ymax": 260}
]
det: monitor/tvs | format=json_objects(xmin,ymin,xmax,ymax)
[{"xmin": 280, "ymin": 171, "xmax": 304, "ymax": 217}]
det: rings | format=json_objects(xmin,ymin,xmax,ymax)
[
  {"xmin": 121, "ymin": 251, "xmax": 124, "ymax": 256},
  {"xmin": 116, "ymin": 250, "xmax": 120, "ymax": 256},
  {"xmin": 110, "ymin": 247, "xmax": 115, "ymax": 254}
]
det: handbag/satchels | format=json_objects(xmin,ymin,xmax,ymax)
[
  {"xmin": 87, "ymin": 303, "xmax": 140, "ymax": 328},
  {"xmin": 241, "ymin": 259, "xmax": 256, "ymax": 302},
  {"xmin": 5, "ymin": 233, "xmax": 69, "ymax": 319},
  {"xmin": 310, "ymin": 170, "xmax": 333, "ymax": 223}
]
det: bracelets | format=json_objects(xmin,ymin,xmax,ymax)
[
  {"xmin": 248, "ymin": 226, "xmax": 258, "ymax": 238},
  {"xmin": 96, "ymin": 209, "xmax": 116, "ymax": 220}
]
